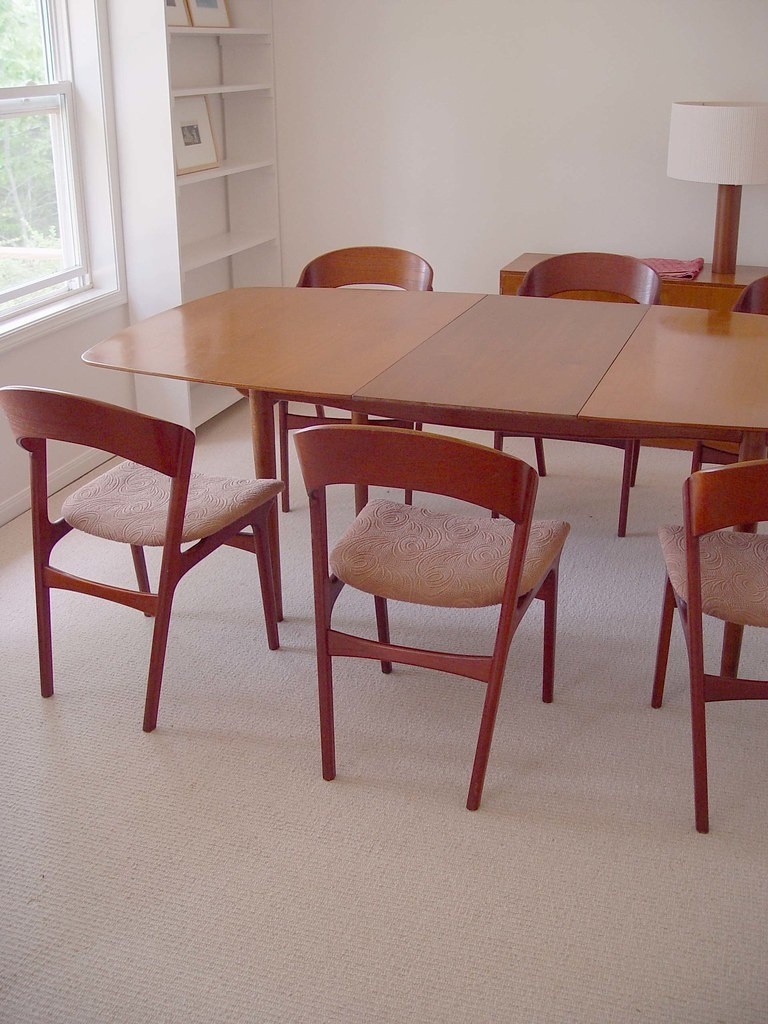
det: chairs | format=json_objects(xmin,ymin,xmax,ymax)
[
  {"xmin": 491, "ymin": 251, "xmax": 662, "ymax": 538},
  {"xmin": 650, "ymin": 457, "xmax": 768, "ymax": 833},
  {"xmin": 278, "ymin": 246, "xmax": 433, "ymax": 512},
  {"xmin": 1, "ymin": 386, "xmax": 285, "ymax": 735},
  {"xmin": 292, "ymin": 424, "xmax": 566, "ymax": 810}
]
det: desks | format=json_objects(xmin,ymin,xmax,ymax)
[{"xmin": 82, "ymin": 286, "xmax": 768, "ymax": 677}]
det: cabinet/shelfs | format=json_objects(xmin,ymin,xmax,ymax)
[
  {"xmin": 498, "ymin": 252, "xmax": 768, "ymax": 453},
  {"xmin": 106, "ymin": 0, "xmax": 279, "ymax": 437}
]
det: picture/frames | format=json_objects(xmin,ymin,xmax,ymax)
[
  {"xmin": 176, "ymin": 95, "xmax": 220, "ymax": 178},
  {"xmin": 166, "ymin": 0, "xmax": 193, "ymax": 27},
  {"xmin": 186, "ymin": 0, "xmax": 233, "ymax": 28}
]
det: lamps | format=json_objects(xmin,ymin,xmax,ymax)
[{"xmin": 667, "ymin": 103, "xmax": 768, "ymax": 274}]
